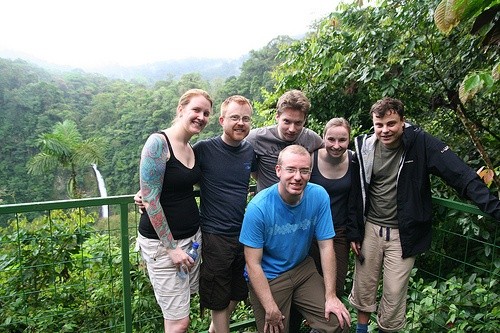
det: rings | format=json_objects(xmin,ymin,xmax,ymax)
[{"xmin": 181, "ymin": 264, "xmax": 186, "ymax": 268}]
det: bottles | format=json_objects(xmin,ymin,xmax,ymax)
[{"xmin": 177, "ymin": 242, "xmax": 199, "ymax": 279}]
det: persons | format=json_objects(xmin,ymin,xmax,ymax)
[
  {"xmin": 239, "ymin": 145, "xmax": 352, "ymax": 333},
  {"xmin": 348, "ymin": 97, "xmax": 500, "ymax": 333},
  {"xmin": 134, "ymin": 95, "xmax": 257, "ymax": 333},
  {"xmin": 309, "ymin": 117, "xmax": 363, "ymax": 297},
  {"xmin": 138, "ymin": 89, "xmax": 213, "ymax": 333},
  {"xmin": 246, "ymin": 88, "xmax": 325, "ymax": 193}
]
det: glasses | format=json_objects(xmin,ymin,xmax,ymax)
[{"xmin": 279, "ymin": 164, "xmax": 313, "ymax": 176}]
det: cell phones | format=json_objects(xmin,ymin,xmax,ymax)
[{"xmin": 356, "ymin": 246, "xmax": 365, "ymax": 265}]
